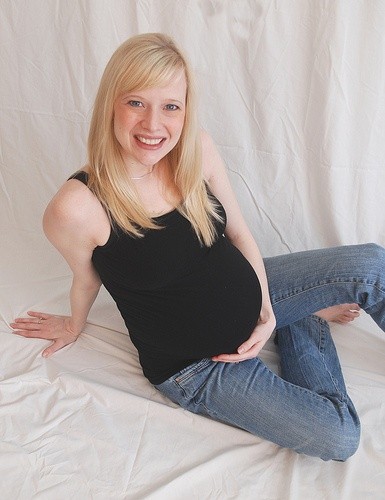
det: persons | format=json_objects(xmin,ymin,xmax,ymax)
[{"xmin": 9, "ymin": 32, "xmax": 385, "ymax": 462}]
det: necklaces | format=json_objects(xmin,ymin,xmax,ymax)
[{"xmin": 130, "ymin": 164, "xmax": 155, "ymax": 180}]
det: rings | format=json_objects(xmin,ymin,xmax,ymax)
[
  {"xmin": 40, "ymin": 313, "xmax": 43, "ymax": 317},
  {"xmin": 39, "ymin": 317, "xmax": 42, "ymax": 323}
]
